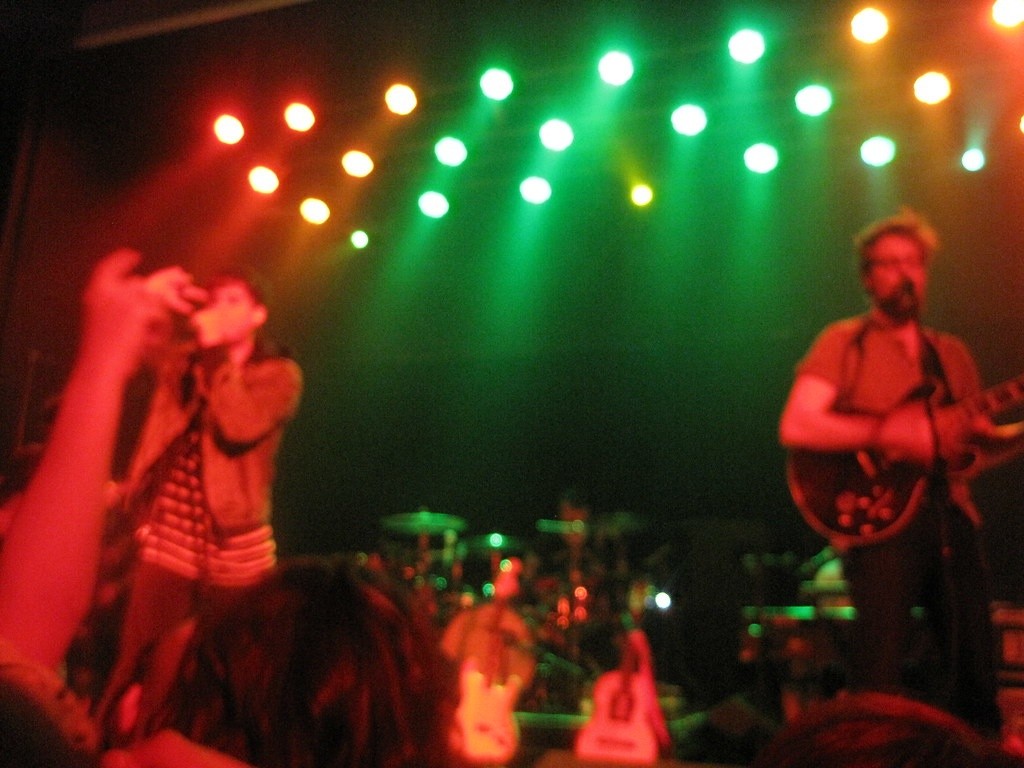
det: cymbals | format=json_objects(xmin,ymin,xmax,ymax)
[{"xmin": 376, "ymin": 503, "xmax": 468, "ymax": 537}]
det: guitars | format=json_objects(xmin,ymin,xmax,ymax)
[
  {"xmin": 780, "ymin": 372, "xmax": 1024, "ymax": 548},
  {"xmin": 571, "ymin": 573, "xmax": 662, "ymax": 766},
  {"xmin": 447, "ymin": 556, "xmax": 528, "ymax": 765}
]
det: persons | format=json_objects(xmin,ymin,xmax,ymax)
[
  {"xmin": 101, "ymin": 569, "xmax": 457, "ymax": 768},
  {"xmin": 779, "ymin": 215, "xmax": 1023, "ymax": 743},
  {"xmin": 0, "ymin": 247, "xmax": 175, "ymax": 673},
  {"xmin": 97, "ymin": 264, "xmax": 303, "ymax": 721}
]
